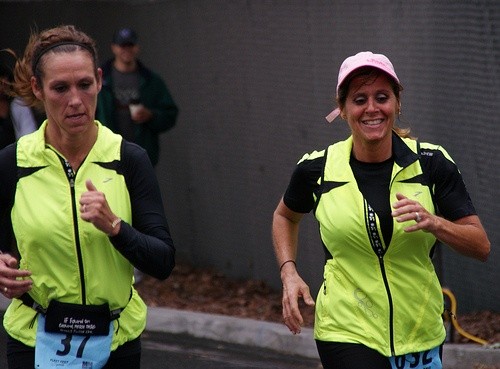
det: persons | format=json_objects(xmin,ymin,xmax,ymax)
[
  {"xmin": 271, "ymin": 51, "xmax": 491, "ymax": 369},
  {"xmin": 0, "ymin": 76, "xmax": 47, "ymax": 150},
  {"xmin": 94, "ymin": 29, "xmax": 180, "ymax": 167},
  {"xmin": 0, "ymin": 26, "xmax": 176, "ymax": 369}
]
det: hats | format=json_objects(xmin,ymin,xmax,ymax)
[
  {"xmin": 113, "ymin": 28, "xmax": 136, "ymax": 46},
  {"xmin": 325, "ymin": 51, "xmax": 404, "ymax": 123}
]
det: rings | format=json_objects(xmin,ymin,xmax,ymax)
[
  {"xmin": 4, "ymin": 287, "xmax": 7, "ymax": 293},
  {"xmin": 83, "ymin": 204, "xmax": 86, "ymax": 212},
  {"xmin": 415, "ymin": 212, "xmax": 421, "ymax": 222}
]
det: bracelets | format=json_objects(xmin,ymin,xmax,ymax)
[
  {"xmin": 280, "ymin": 260, "xmax": 296, "ymax": 271},
  {"xmin": 110, "ymin": 218, "xmax": 121, "ymax": 235}
]
